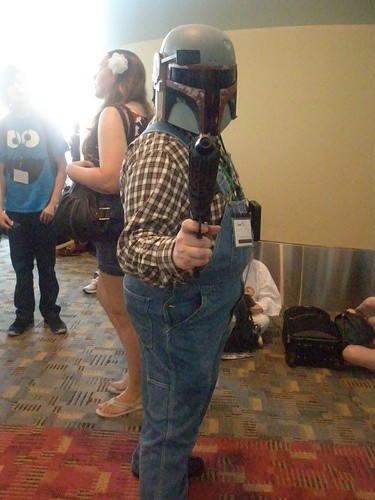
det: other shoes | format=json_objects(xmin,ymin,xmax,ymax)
[{"xmin": 129, "ymin": 451, "xmax": 206, "ymax": 479}]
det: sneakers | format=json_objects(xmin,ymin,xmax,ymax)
[
  {"xmin": 3, "ymin": 310, "xmax": 34, "ymax": 338},
  {"xmin": 82, "ymin": 275, "xmax": 98, "ymax": 294},
  {"xmin": 40, "ymin": 307, "xmax": 67, "ymax": 335}
]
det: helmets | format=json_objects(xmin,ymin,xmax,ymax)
[{"xmin": 151, "ymin": 25, "xmax": 239, "ymax": 136}]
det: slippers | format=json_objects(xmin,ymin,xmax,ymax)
[{"xmin": 95, "ymin": 380, "xmax": 143, "ymax": 418}]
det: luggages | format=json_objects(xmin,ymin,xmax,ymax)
[{"xmin": 282, "ymin": 305, "xmax": 346, "ymax": 371}]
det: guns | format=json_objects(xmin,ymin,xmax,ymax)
[{"xmin": 188, "ymin": 133, "xmax": 220, "ymax": 278}]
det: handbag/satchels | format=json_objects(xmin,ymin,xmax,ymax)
[{"xmin": 51, "ymin": 183, "xmax": 107, "ymax": 243}]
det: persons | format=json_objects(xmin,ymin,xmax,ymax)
[
  {"xmin": 0, "ymin": 90, "xmax": 98, "ymax": 337},
  {"xmin": 224, "ymin": 257, "xmax": 283, "ymax": 350},
  {"xmin": 65, "ymin": 50, "xmax": 155, "ymax": 418},
  {"xmin": 116, "ymin": 23, "xmax": 254, "ymax": 500},
  {"xmin": 343, "ymin": 296, "xmax": 375, "ymax": 373}
]
